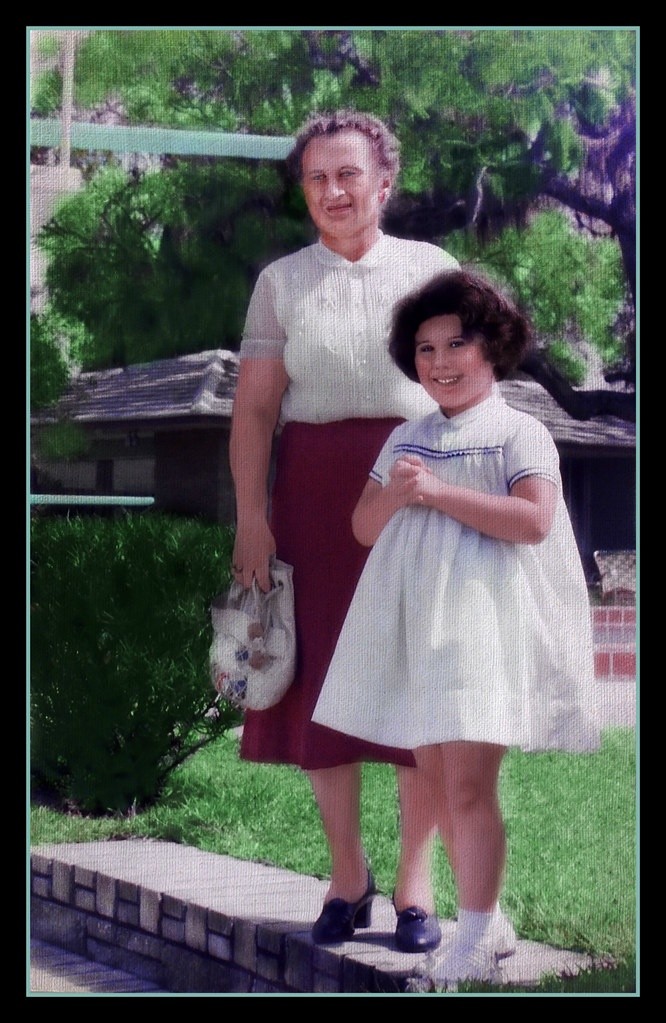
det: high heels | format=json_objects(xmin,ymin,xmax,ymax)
[
  {"xmin": 392, "ymin": 886, "xmax": 442, "ymax": 952},
  {"xmin": 311, "ymin": 859, "xmax": 375, "ymax": 946}
]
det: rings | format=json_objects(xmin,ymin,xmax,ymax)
[{"xmin": 233, "ymin": 564, "xmax": 243, "ymax": 574}]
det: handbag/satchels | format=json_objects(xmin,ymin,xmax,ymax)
[{"xmin": 209, "ymin": 557, "xmax": 297, "ymax": 709}]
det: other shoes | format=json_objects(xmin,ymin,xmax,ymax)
[{"xmin": 430, "ymin": 901, "xmax": 517, "ymax": 984}]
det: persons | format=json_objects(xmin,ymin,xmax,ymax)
[
  {"xmin": 311, "ymin": 271, "xmax": 600, "ymax": 979},
  {"xmin": 229, "ymin": 112, "xmax": 463, "ymax": 952}
]
